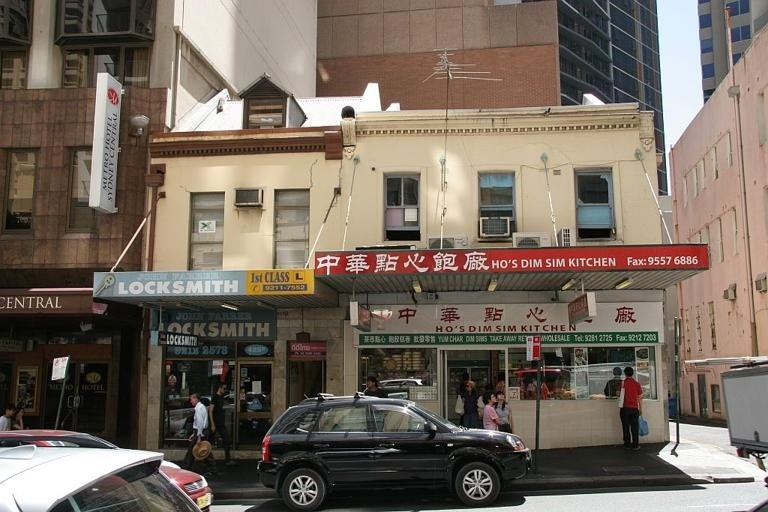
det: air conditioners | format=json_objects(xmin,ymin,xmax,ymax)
[
  {"xmin": 512, "ymin": 227, "xmax": 574, "ymax": 248},
  {"xmin": 755, "ymin": 278, "xmax": 766, "ymax": 291},
  {"xmin": 723, "ymin": 289, "xmax": 734, "ymax": 300},
  {"xmin": 427, "ymin": 237, "xmax": 455, "ymax": 250},
  {"xmin": 478, "ymin": 216, "xmax": 511, "ymax": 238},
  {"xmin": 232, "ymin": 187, "xmax": 263, "ymax": 208}
]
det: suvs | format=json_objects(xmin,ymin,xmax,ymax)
[{"xmin": 259, "ymin": 392, "xmax": 531, "ymax": 511}]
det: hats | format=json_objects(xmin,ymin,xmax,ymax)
[
  {"xmin": 465, "ymin": 384, "xmax": 470, "ymax": 387},
  {"xmin": 192, "ymin": 439, "xmax": 212, "ymax": 462}
]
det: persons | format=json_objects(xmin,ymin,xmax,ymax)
[
  {"xmin": 0, "ymin": 403, "xmax": 24, "ymax": 430},
  {"xmin": 461, "ymin": 379, "xmax": 479, "ymax": 429},
  {"xmin": 181, "ymin": 394, "xmax": 217, "ymax": 472},
  {"xmin": 209, "ymin": 383, "xmax": 237, "ymax": 467},
  {"xmin": 604, "ymin": 367, "xmax": 623, "ymax": 396},
  {"xmin": 482, "ymin": 392, "xmax": 514, "ymax": 434},
  {"xmin": 363, "ymin": 376, "xmax": 389, "ymax": 398},
  {"xmin": 527, "ymin": 375, "xmax": 549, "ymax": 400},
  {"xmin": 618, "ymin": 367, "xmax": 649, "ymax": 451}
]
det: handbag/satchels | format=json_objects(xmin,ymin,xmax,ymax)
[
  {"xmin": 617, "ymin": 380, "xmax": 626, "ymax": 408},
  {"xmin": 455, "ymin": 394, "xmax": 465, "ymax": 416},
  {"xmin": 638, "ymin": 415, "xmax": 649, "ymax": 436}
]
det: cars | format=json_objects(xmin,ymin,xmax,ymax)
[{"xmin": 1, "ymin": 429, "xmax": 213, "ymax": 511}]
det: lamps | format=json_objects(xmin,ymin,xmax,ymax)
[
  {"xmin": 412, "ymin": 279, "xmax": 423, "ymax": 294},
  {"xmin": 129, "ymin": 112, "xmax": 151, "ymax": 137},
  {"xmin": 615, "ymin": 278, "xmax": 633, "ymax": 290},
  {"xmin": 487, "ymin": 278, "xmax": 500, "ymax": 293},
  {"xmin": 561, "ymin": 278, "xmax": 577, "ymax": 293}
]
